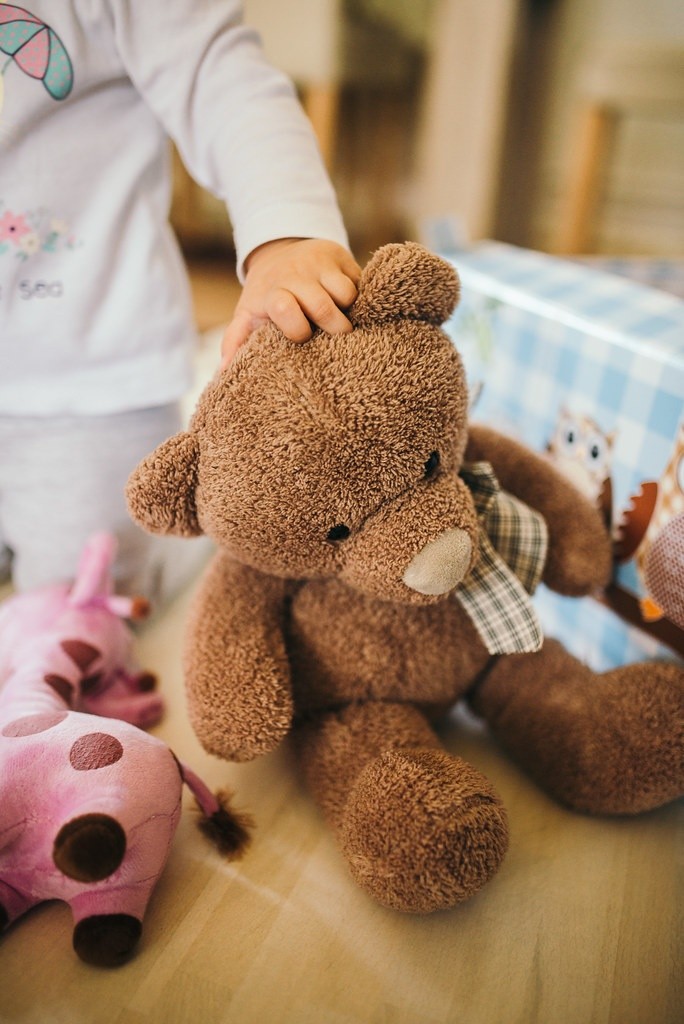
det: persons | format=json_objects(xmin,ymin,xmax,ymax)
[{"xmin": 0, "ymin": 0, "xmax": 364, "ymax": 631}]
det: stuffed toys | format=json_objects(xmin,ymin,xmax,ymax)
[
  {"xmin": 128, "ymin": 241, "xmax": 684, "ymax": 915},
  {"xmin": 0, "ymin": 529, "xmax": 252, "ymax": 969}
]
